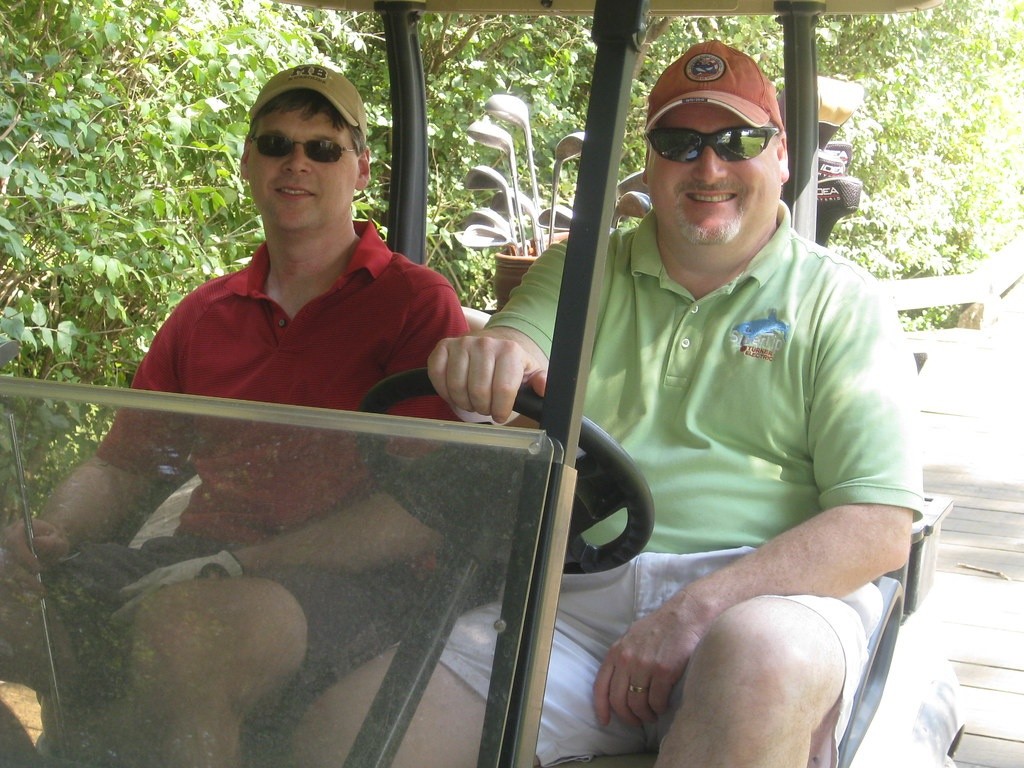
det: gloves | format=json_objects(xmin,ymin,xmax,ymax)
[{"xmin": 110, "ymin": 548, "xmax": 244, "ymax": 630}]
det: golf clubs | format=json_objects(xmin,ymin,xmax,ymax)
[
  {"xmin": 0, "ymin": 340, "xmax": 77, "ymax": 768},
  {"xmin": 449, "ymin": 90, "xmax": 653, "ymax": 255}
]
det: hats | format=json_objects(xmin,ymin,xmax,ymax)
[
  {"xmin": 250, "ymin": 64, "xmax": 368, "ymax": 143},
  {"xmin": 645, "ymin": 39, "xmax": 785, "ymax": 140}
]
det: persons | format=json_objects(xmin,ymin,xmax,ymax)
[
  {"xmin": 0, "ymin": 60, "xmax": 473, "ymax": 768},
  {"xmin": 291, "ymin": 37, "xmax": 930, "ymax": 768}
]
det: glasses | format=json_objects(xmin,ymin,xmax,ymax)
[
  {"xmin": 645, "ymin": 125, "xmax": 780, "ymax": 163},
  {"xmin": 250, "ymin": 133, "xmax": 358, "ymax": 163}
]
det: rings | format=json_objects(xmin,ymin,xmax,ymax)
[{"xmin": 629, "ymin": 683, "xmax": 649, "ymax": 697}]
